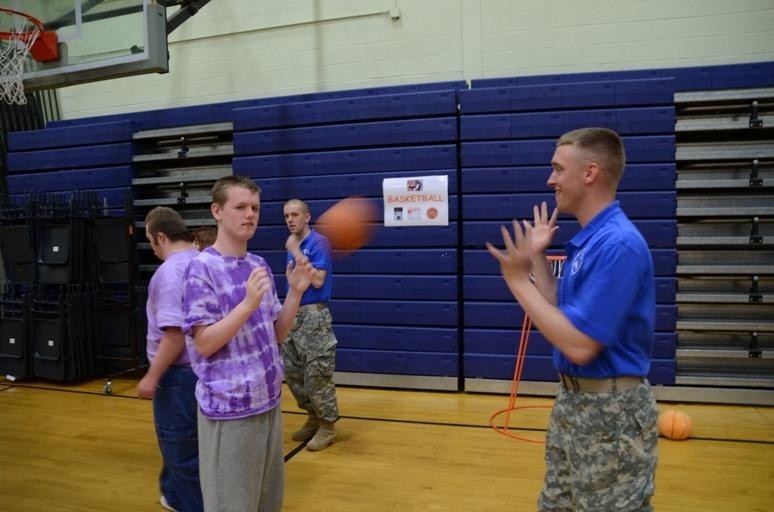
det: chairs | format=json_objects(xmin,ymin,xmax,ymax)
[{"xmin": 0, "ymin": 189, "xmax": 137, "ymax": 384}]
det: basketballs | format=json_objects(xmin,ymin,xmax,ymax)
[
  {"xmin": 658, "ymin": 407, "xmax": 691, "ymax": 441},
  {"xmin": 313, "ymin": 198, "xmax": 378, "ymax": 254}
]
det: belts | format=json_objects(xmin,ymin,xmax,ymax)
[{"xmin": 558, "ymin": 372, "xmax": 641, "ymax": 393}]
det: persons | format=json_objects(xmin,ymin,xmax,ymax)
[
  {"xmin": 191, "ymin": 226, "xmax": 218, "ymax": 253},
  {"xmin": 485, "ymin": 123, "xmax": 659, "ymax": 511},
  {"xmin": 280, "ymin": 195, "xmax": 340, "ymax": 451},
  {"xmin": 180, "ymin": 175, "xmax": 315, "ymax": 512},
  {"xmin": 137, "ymin": 204, "xmax": 203, "ymax": 511}
]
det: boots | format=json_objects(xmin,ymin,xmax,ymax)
[
  {"xmin": 306, "ymin": 419, "xmax": 337, "ymax": 451},
  {"xmin": 292, "ymin": 412, "xmax": 319, "ymax": 441}
]
